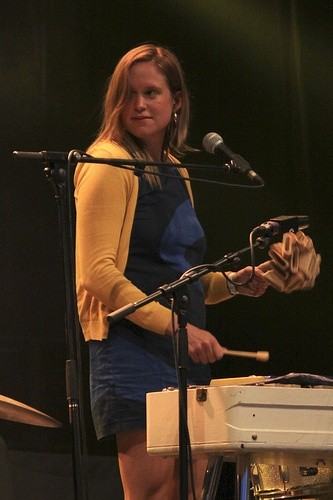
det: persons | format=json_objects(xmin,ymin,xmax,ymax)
[{"xmin": 73, "ymin": 44, "xmax": 270, "ymax": 500}]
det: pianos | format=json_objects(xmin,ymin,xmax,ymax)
[{"xmin": 145, "ymin": 384, "xmax": 333, "ymax": 460}]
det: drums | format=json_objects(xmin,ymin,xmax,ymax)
[{"xmin": 250, "ymin": 460, "xmax": 333, "ymax": 500}]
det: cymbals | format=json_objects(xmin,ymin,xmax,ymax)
[{"xmin": 0, "ymin": 394, "xmax": 63, "ymax": 428}]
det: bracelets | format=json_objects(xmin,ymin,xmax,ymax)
[
  {"xmin": 170, "ymin": 328, "xmax": 179, "ymax": 340},
  {"xmin": 228, "ymin": 272, "xmax": 238, "ymax": 295}
]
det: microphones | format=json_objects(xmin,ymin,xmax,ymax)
[
  {"xmin": 259, "ymin": 215, "xmax": 310, "ymax": 237},
  {"xmin": 202, "ymin": 133, "xmax": 265, "ymax": 185}
]
